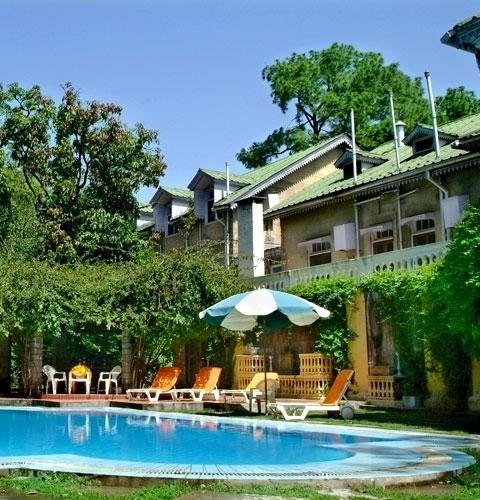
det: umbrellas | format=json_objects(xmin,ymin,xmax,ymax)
[{"xmin": 197, "ymin": 283, "xmax": 331, "ymax": 417}]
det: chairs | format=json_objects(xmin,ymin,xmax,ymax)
[
  {"xmin": 42, "ymin": 362, "xmax": 122, "ymax": 394},
  {"xmin": 125, "ymin": 365, "xmax": 356, "ymax": 421}
]
